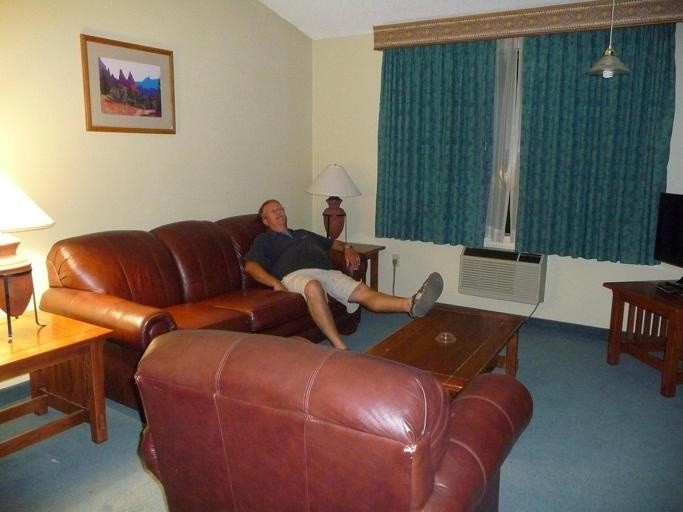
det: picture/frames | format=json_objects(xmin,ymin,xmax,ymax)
[{"xmin": 79, "ymin": 33, "xmax": 177, "ymax": 134}]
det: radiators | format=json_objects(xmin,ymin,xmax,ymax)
[{"xmin": 458, "ymin": 247, "xmax": 547, "ymax": 306}]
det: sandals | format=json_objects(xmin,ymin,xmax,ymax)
[{"xmin": 408, "ymin": 272, "xmax": 443, "ymax": 320}]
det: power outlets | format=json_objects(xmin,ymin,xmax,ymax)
[{"xmin": 392, "ymin": 253, "xmax": 400, "ymax": 267}]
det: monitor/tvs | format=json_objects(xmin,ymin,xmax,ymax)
[{"xmin": 652, "ymin": 191, "xmax": 683, "ymax": 288}]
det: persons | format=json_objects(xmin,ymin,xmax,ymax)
[{"xmin": 243, "ymin": 199, "xmax": 443, "ymax": 351}]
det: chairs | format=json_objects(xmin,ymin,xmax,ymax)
[{"xmin": 132, "ymin": 330, "xmax": 534, "ymax": 512}]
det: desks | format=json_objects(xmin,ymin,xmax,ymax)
[
  {"xmin": 0, "ymin": 308, "xmax": 115, "ymax": 456},
  {"xmin": 602, "ymin": 279, "xmax": 683, "ymax": 397},
  {"xmin": 339, "ymin": 242, "xmax": 386, "ymax": 322}
]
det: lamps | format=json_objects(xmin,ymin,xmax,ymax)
[
  {"xmin": 306, "ymin": 163, "xmax": 363, "ymax": 238},
  {"xmin": 588, "ymin": 0, "xmax": 631, "ymax": 82},
  {"xmin": 0, "ymin": 183, "xmax": 56, "ymax": 338}
]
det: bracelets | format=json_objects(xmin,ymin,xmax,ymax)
[{"xmin": 342, "ymin": 245, "xmax": 352, "ymax": 253}]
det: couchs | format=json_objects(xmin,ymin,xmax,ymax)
[{"xmin": 41, "ymin": 213, "xmax": 367, "ymax": 411}]
